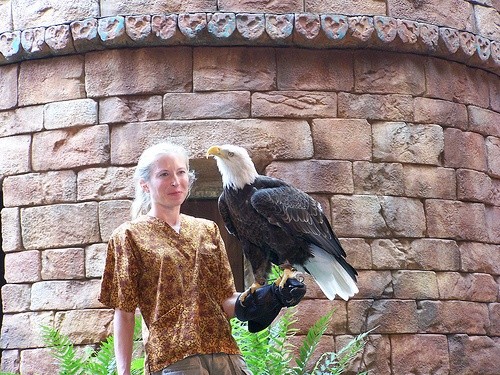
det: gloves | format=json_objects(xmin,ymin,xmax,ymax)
[{"xmin": 235, "ymin": 277, "xmax": 308, "ymax": 333}]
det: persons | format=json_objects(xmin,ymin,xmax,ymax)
[{"xmin": 98, "ymin": 143, "xmax": 306, "ymax": 375}]
